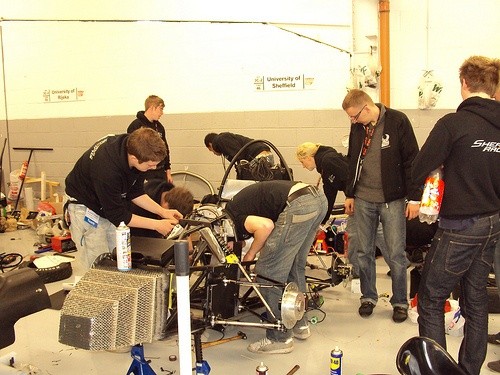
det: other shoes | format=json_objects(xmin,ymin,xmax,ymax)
[
  {"xmin": 487, "ymin": 335, "xmax": 500, "ymax": 344},
  {"xmin": 393, "ymin": 307, "xmax": 406, "ymax": 321},
  {"xmin": 487, "ymin": 360, "xmax": 500, "ymax": 373},
  {"xmin": 359, "ymin": 301, "xmax": 376, "ymax": 315}
]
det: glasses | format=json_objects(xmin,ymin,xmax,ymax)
[{"xmin": 348, "ymin": 105, "xmax": 368, "ymax": 123}]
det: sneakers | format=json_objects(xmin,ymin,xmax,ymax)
[
  {"xmin": 294, "ymin": 325, "xmax": 312, "ymax": 339},
  {"xmin": 247, "ymin": 339, "xmax": 293, "ymax": 354}
]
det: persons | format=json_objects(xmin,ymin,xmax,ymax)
[
  {"xmin": 126, "ymin": 95, "xmax": 174, "ymax": 184},
  {"xmin": 296, "ymin": 142, "xmax": 348, "ymax": 225},
  {"xmin": 408, "ymin": 56, "xmax": 500, "ymax": 375},
  {"xmin": 222, "ymin": 180, "xmax": 328, "ymax": 354},
  {"xmin": 128, "ymin": 178, "xmax": 194, "ymax": 239},
  {"xmin": 342, "ymin": 89, "xmax": 421, "ymax": 323},
  {"xmin": 63, "ymin": 127, "xmax": 168, "ymax": 270},
  {"xmin": 204, "ymin": 132, "xmax": 274, "ymax": 182},
  {"xmin": 488, "ymin": 59, "xmax": 500, "ymax": 373}
]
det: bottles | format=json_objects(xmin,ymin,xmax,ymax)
[
  {"xmin": 18, "ymin": 160, "xmax": 29, "ymax": 180},
  {"xmin": 418, "ymin": 164, "xmax": 445, "ymax": 225}
]
